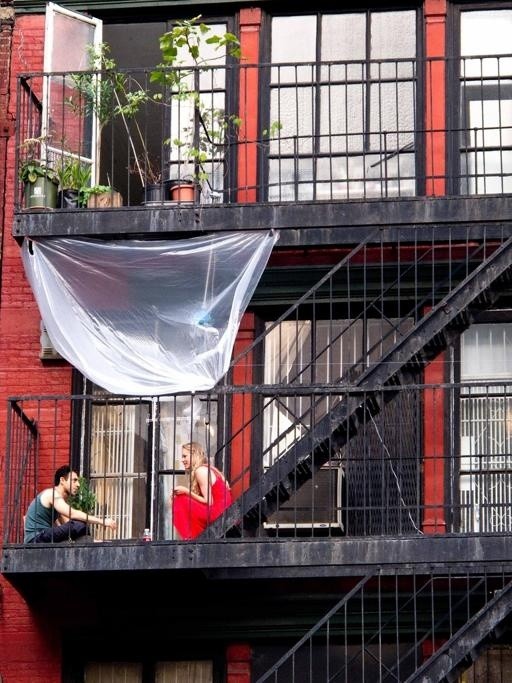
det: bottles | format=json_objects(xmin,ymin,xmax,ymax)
[{"xmin": 142, "ymin": 528, "xmax": 152, "ymax": 542}]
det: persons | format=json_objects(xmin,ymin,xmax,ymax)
[
  {"xmin": 174, "ymin": 442, "xmax": 232, "ymax": 541},
  {"xmin": 24, "ymin": 466, "xmax": 117, "ymax": 543}
]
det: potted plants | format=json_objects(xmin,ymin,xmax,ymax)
[
  {"xmin": 149, "ymin": 14, "xmax": 283, "ymax": 201},
  {"xmin": 59, "ymin": 162, "xmax": 92, "ymax": 209},
  {"xmin": 62, "ymin": 45, "xmax": 146, "ymax": 208},
  {"xmin": 17, "ymin": 133, "xmax": 59, "ymax": 210}
]
